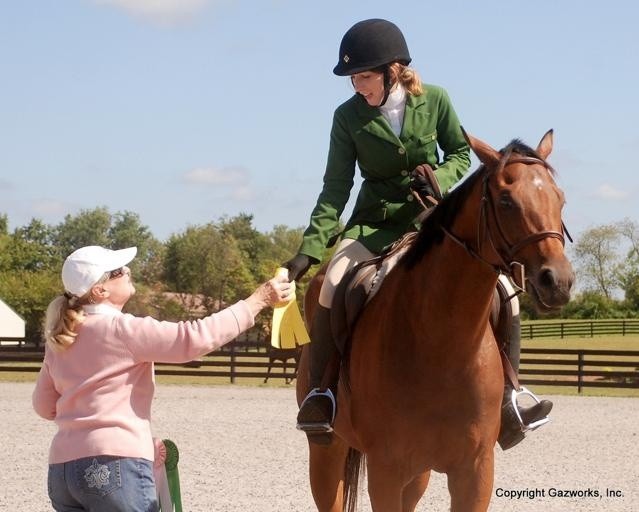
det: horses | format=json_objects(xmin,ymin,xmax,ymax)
[
  {"xmin": 259, "ymin": 332, "xmax": 304, "ymax": 384},
  {"xmin": 297, "ymin": 124, "xmax": 576, "ymax": 512}
]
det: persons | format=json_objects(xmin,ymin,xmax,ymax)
[
  {"xmin": 32, "ymin": 246, "xmax": 296, "ymax": 512},
  {"xmin": 281, "ymin": 19, "xmax": 552, "ymax": 451}
]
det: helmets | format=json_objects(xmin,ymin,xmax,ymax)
[{"xmin": 332, "ymin": 19, "xmax": 412, "ymax": 76}]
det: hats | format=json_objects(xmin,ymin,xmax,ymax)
[{"xmin": 61, "ymin": 244, "xmax": 137, "ymax": 300}]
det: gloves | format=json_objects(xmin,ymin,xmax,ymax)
[
  {"xmin": 280, "ymin": 254, "xmax": 313, "ymax": 284},
  {"xmin": 408, "ymin": 174, "xmax": 436, "ymax": 200}
]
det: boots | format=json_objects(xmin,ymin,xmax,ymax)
[
  {"xmin": 297, "ymin": 301, "xmax": 335, "ymax": 445},
  {"xmin": 497, "ymin": 313, "xmax": 553, "ymax": 451}
]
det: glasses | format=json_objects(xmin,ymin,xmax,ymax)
[{"xmin": 108, "ymin": 268, "xmax": 124, "ymax": 281}]
